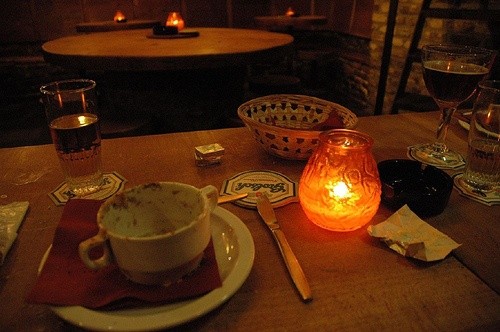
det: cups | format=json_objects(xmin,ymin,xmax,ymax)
[
  {"xmin": 462, "ymin": 79, "xmax": 500, "ymax": 193},
  {"xmin": 166, "ymin": 12, "xmax": 185, "ymax": 34},
  {"xmin": 298, "ymin": 128, "xmax": 382, "ymax": 233},
  {"xmin": 40, "ymin": 79, "xmax": 104, "ymax": 197},
  {"xmin": 114, "ymin": 10, "xmax": 125, "ymax": 23},
  {"xmin": 78, "ymin": 181, "xmax": 219, "ymax": 288}
]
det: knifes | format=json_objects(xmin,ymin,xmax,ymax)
[
  {"xmin": 257, "ymin": 194, "xmax": 313, "ymax": 302},
  {"xmin": 215, "ymin": 193, "xmax": 248, "ymax": 204}
]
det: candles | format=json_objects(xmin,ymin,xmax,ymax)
[
  {"xmin": 297, "ymin": 129, "xmax": 381, "ymax": 234},
  {"xmin": 166, "ymin": 11, "xmax": 184, "ymax": 29}
]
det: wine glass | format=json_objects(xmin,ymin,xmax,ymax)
[{"xmin": 409, "ymin": 42, "xmax": 497, "ymax": 172}]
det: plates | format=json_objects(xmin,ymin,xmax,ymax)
[{"xmin": 37, "ymin": 205, "xmax": 255, "ymax": 332}]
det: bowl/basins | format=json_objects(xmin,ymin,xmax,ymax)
[{"xmin": 377, "ymin": 158, "xmax": 454, "ymax": 220}]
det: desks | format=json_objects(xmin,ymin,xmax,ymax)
[
  {"xmin": 255, "ymin": 16, "xmax": 329, "ymax": 35},
  {"xmin": 41, "ymin": 27, "xmax": 295, "ymax": 88},
  {"xmin": 0, "ymin": 111, "xmax": 500, "ymax": 332},
  {"xmin": 76, "ymin": 18, "xmax": 162, "ymax": 31}
]
